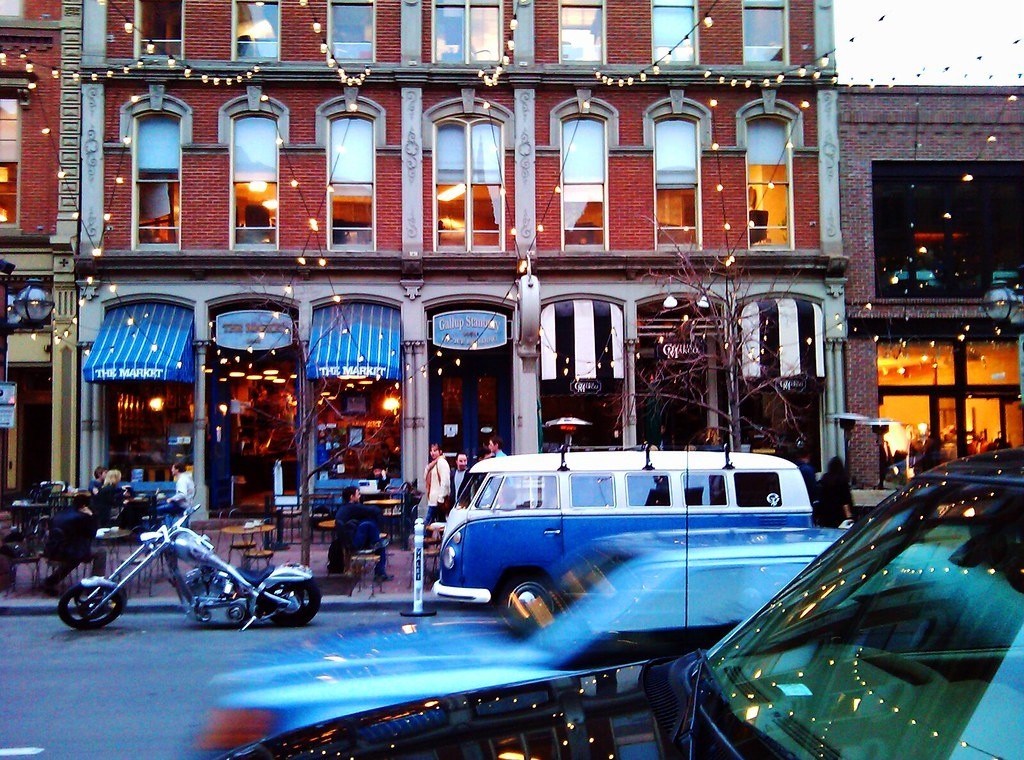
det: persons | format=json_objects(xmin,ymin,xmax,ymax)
[
  {"xmin": 882, "ymin": 422, "xmax": 930, "ymax": 481},
  {"xmin": 366, "ymin": 464, "xmax": 390, "ymax": 491},
  {"xmin": 162, "ymin": 461, "xmax": 194, "ymax": 529},
  {"xmin": 332, "ymin": 486, "xmax": 394, "ymax": 582},
  {"xmin": 941, "ymin": 424, "xmax": 1003, "ymax": 462},
  {"xmin": 659, "ymin": 425, "xmax": 670, "ymax": 451},
  {"xmin": 791, "ymin": 449, "xmax": 853, "ymax": 529},
  {"xmin": 43, "ymin": 465, "xmax": 131, "ymax": 594},
  {"xmin": 415, "ymin": 437, "xmax": 516, "ymax": 549}
]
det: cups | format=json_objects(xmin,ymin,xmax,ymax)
[
  {"xmin": 253, "ymin": 519, "xmax": 260, "ymax": 525},
  {"xmin": 111, "ymin": 526, "xmax": 119, "ymax": 532},
  {"xmin": 245, "ymin": 522, "xmax": 253, "ymax": 527}
]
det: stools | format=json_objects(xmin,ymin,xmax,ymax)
[{"xmin": 7, "ymin": 527, "xmax": 44, "ymax": 595}]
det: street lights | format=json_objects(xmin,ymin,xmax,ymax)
[{"xmin": 0, "ymin": 275, "xmax": 58, "ymax": 515}]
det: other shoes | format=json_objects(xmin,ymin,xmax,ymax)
[
  {"xmin": 374, "ymin": 575, "xmax": 395, "ymax": 583},
  {"xmin": 371, "ymin": 538, "xmax": 389, "ymax": 549}
]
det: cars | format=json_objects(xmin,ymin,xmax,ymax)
[{"xmin": 190, "ymin": 523, "xmax": 918, "ymax": 760}]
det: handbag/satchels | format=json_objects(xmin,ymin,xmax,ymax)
[{"xmin": 437, "ymin": 495, "xmax": 450, "ymax": 513}]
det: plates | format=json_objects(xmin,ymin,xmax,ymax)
[
  {"xmin": 254, "ymin": 523, "xmax": 264, "ymax": 526},
  {"xmin": 243, "ymin": 525, "xmax": 254, "ymax": 528}
]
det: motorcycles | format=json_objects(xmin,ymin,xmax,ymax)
[{"xmin": 57, "ymin": 500, "xmax": 323, "ymax": 631}]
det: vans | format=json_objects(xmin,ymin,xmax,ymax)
[{"xmin": 428, "ymin": 446, "xmax": 819, "ymax": 637}]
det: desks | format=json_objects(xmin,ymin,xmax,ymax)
[
  {"xmin": 366, "ymin": 499, "xmax": 405, "ymax": 543},
  {"xmin": 64, "ymin": 490, "xmax": 91, "ymax": 500},
  {"xmin": 9, "ymin": 503, "xmax": 51, "ymax": 532},
  {"xmin": 318, "ymin": 518, "xmax": 338, "ymax": 529},
  {"xmin": 95, "ymin": 530, "xmax": 131, "ymax": 576},
  {"xmin": 220, "ymin": 521, "xmax": 276, "ymax": 568}
]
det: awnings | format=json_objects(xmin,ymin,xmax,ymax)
[
  {"xmin": 82, "ymin": 302, "xmax": 195, "ymax": 385},
  {"xmin": 307, "ymin": 302, "xmax": 401, "ymax": 383}
]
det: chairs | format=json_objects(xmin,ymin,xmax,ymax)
[
  {"xmin": 329, "ymin": 520, "xmax": 382, "ymax": 596},
  {"xmin": 115, "ymin": 522, "xmax": 157, "ymax": 602},
  {"xmin": 408, "ymin": 523, "xmax": 441, "ymax": 588},
  {"xmin": 244, "ymin": 519, "xmax": 276, "ymax": 570},
  {"xmin": 410, "ymin": 504, "xmax": 441, "ymax": 574},
  {"xmin": 44, "ymin": 527, "xmax": 79, "ymax": 594},
  {"xmin": 230, "ymin": 508, "xmax": 258, "ymax": 568}
]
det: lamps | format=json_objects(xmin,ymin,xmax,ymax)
[
  {"xmin": 437, "ymin": 185, "xmax": 468, "ymax": 205},
  {"xmin": 247, "ymin": 181, "xmax": 267, "ymax": 194},
  {"xmin": 260, "ymin": 199, "xmax": 278, "ymax": 211}
]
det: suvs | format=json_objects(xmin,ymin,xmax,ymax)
[{"xmin": 209, "ymin": 447, "xmax": 1024, "ymax": 760}]
